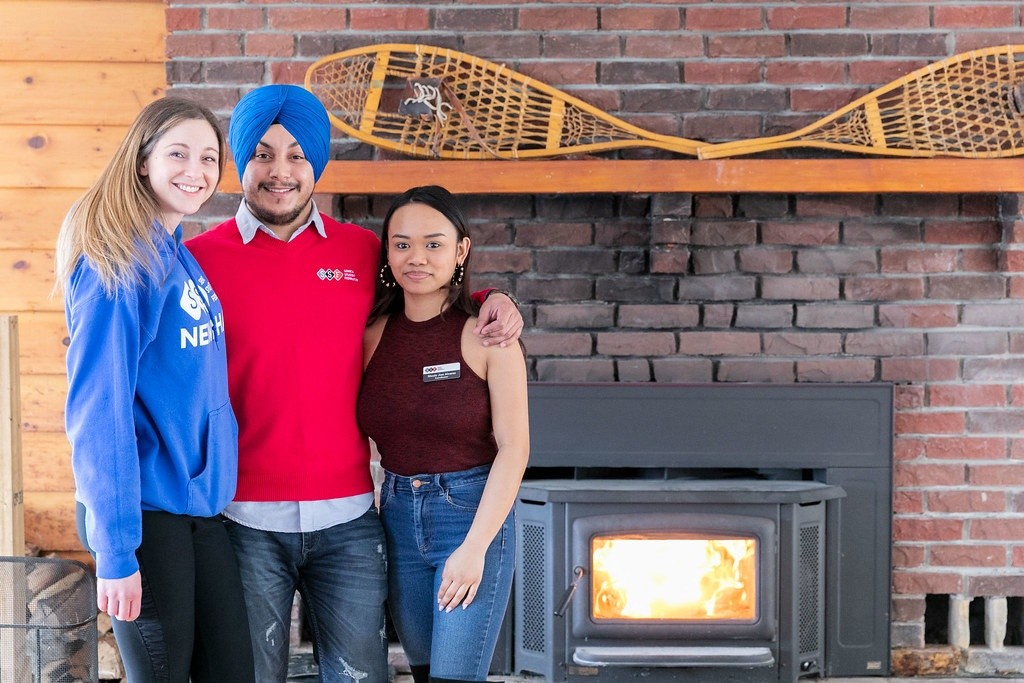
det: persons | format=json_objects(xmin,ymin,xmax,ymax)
[
  {"xmin": 55, "ymin": 97, "xmax": 256, "ymax": 683},
  {"xmin": 356, "ymin": 184, "xmax": 531, "ymax": 683},
  {"xmin": 63, "ymin": 84, "xmax": 524, "ymax": 683}
]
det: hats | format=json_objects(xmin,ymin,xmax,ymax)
[{"xmin": 228, "ymin": 84, "xmax": 331, "ymax": 184}]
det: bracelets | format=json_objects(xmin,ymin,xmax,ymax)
[{"xmin": 489, "ymin": 289, "xmax": 521, "ymax": 309}]
